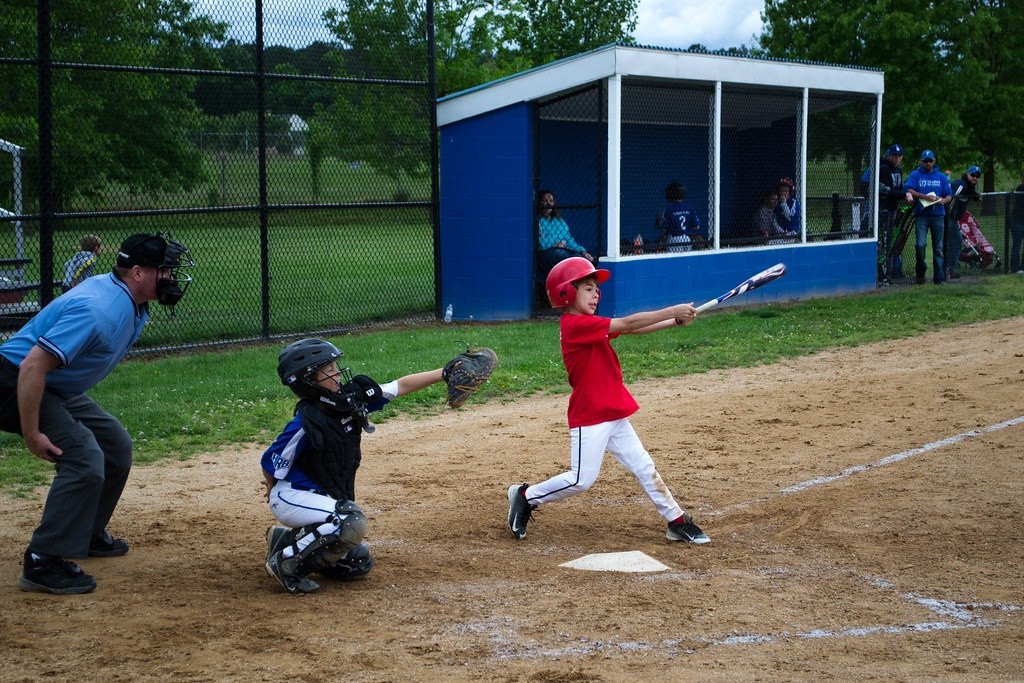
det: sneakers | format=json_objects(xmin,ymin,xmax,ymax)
[
  {"xmin": 666, "ymin": 515, "xmax": 712, "ymax": 544},
  {"xmin": 86, "ymin": 533, "xmax": 129, "ymax": 556},
  {"xmin": 507, "ymin": 483, "xmax": 541, "ymax": 541},
  {"xmin": 17, "ymin": 549, "xmax": 96, "ymax": 594},
  {"xmin": 265, "ymin": 551, "xmax": 320, "ymax": 592},
  {"xmin": 266, "ymin": 526, "xmax": 292, "ymax": 559}
]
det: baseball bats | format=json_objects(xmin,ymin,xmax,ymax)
[{"xmin": 675, "ymin": 262, "xmax": 788, "ymax": 324}]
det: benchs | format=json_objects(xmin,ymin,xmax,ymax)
[{"xmin": 0, "ymin": 257, "xmax": 64, "ymax": 332}]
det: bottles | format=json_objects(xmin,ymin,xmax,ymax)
[{"xmin": 633, "ymin": 234, "xmax": 644, "ymax": 258}]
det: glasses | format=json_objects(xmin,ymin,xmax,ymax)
[
  {"xmin": 923, "ymin": 159, "xmax": 935, "ymax": 163},
  {"xmin": 970, "ymin": 173, "xmax": 981, "ymax": 178}
]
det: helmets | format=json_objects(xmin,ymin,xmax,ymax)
[
  {"xmin": 546, "ymin": 257, "xmax": 610, "ymax": 308},
  {"xmin": 663, "ymin": 182, "xmax": 687, "ymax": 202},
  {"xmin": 778, "ymin": 177, "xmax": 794, "ymax": 190},
  {"xmin": 277, "ymin": 337, "xmax": 361, "ymax": 410}
]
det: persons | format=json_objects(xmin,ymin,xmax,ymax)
[
  {"xmin": 656, "ymin": 182, "xmax": 701, "ymax": 252},
  {"xmin": 0, "ymin": 233, "xmax": 193, "ymax": 595},
  {"xmin": 1008, "ymin": 170, "xmax": 1024, "ymax": 273},
  {"xmin": 259, "ymin": 337, "xmax": 499, "ymax": 594},
  {"xmin": 752, "ymin": 177, "xmax": 803, "ymax": 245},
  {"xmin": 506, "ymin": 257, "xmax": 712, "ymax": 546},
  {"xmin": 536, "ymin": 190, "xmax": 595, "ymax": 268},
  {"xmin": 858, "ymin": 143, "xmax": 983, "ymax": 289}
]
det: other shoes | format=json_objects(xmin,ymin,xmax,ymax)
[
  {"xmin": 916, "ymin": 273, "xmax": 925, "ymax": 284},
  {"xmin": 1011, "ymin": 267, "xmax": 1024, "ymax": 274},
  {"xmin": 937, "ymin": 280, "xmax": 945, "ymax": 285}
]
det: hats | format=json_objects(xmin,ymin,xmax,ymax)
[
  {"xmin": 117, "ymin": 234, "xmax": 181, "ymax": 270},
  {"xmin": 889, "ymin": 144, "xmax": 903, "ymax": 155},
  {"xmin": 920, "ymin": 150, "xmax": 936, "ymax": 163},
  {"xmin": 967, "ymin": 166, "xmax": 983, "ymax": 176}
]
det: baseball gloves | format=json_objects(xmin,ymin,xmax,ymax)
[{"xmin": 441, "ymin": 347, "xmax": 498, "ymax": 408}]
